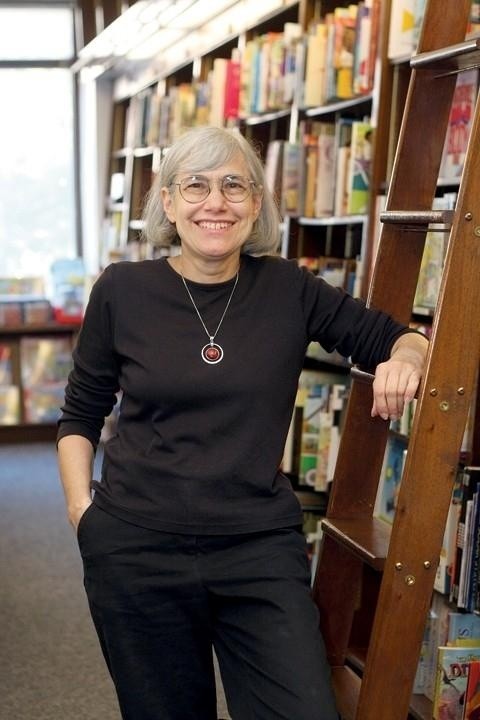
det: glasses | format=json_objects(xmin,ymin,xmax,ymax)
[{"xmin": 169, "ymin": 173, "xmax": 263, "ymax": 205}]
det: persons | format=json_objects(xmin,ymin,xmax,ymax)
[{"xmin": 54, "ymin": 127, "xmax": 431, "ymax": 719}]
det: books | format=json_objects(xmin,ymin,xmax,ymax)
[
  {"xmin": 103, "ymin": 1, "xmax": 480, "ymax": 359},
  {"xmin": 279, "ymin": 369, "xmax": 480, "ymax": 719},
  {"xmin": 0, "ymin": 256, "xmax": 104, "ymax": 426}
]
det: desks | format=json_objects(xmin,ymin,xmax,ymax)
[{"xmin": 0, "ymin": 273, "xmax": 83, "ymax": 446}]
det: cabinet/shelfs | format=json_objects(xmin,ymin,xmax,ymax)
[{"xmin": 94, "ymin": 0, "xmax": 480, "ymax": 720}]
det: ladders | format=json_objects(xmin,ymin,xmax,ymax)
[{"xmin": 311, "ymin": 0, "xmax": 476, "ymax": 716}]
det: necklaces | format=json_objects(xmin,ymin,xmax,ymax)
[{"xmin": 175, "ymin": 255, "xmax": 238, "ymax": 364}]
